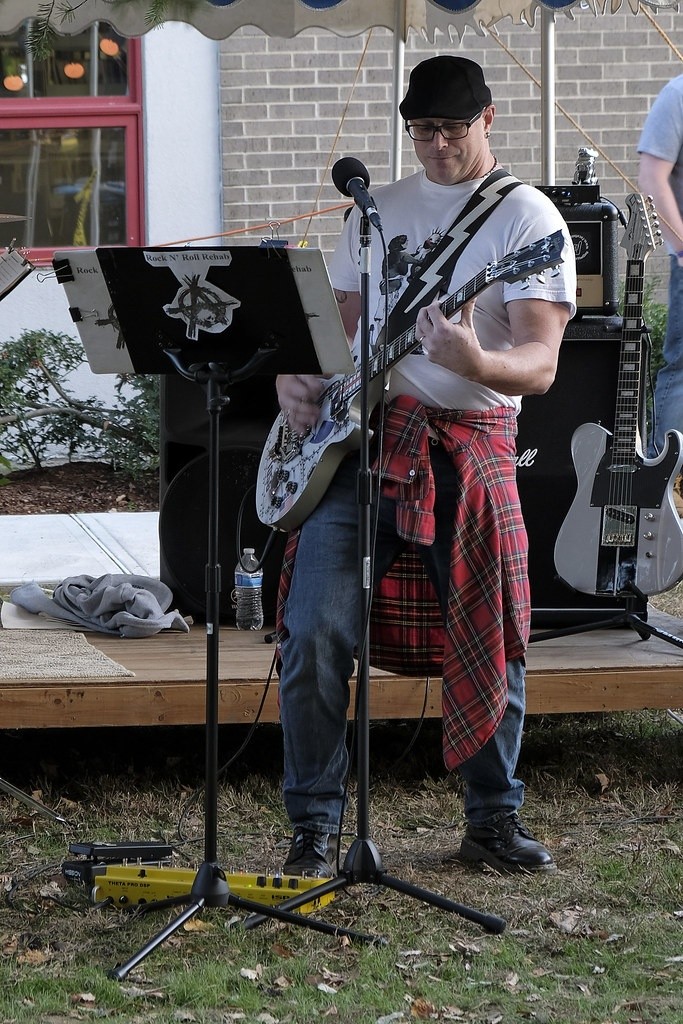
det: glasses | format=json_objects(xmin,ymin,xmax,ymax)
[{"xmin": 405, "ymin": 106, "xmax": 488, "ymax": 141}]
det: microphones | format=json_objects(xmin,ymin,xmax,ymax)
[{"xmin": 332, "ymin": 157, "xmax": 383, "ymax": 233}]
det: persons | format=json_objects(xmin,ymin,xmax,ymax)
[
  {"xmin": 274, "ymin": 54, "xmax": 578, "ymax": 881},
  {"xmin": 635, "ymin": 70, "xmax": 682, "ymax": 463}
]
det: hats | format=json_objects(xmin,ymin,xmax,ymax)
[{"xmin": 399, "ymin": 55, "xmax": 491, "ymax": 120}]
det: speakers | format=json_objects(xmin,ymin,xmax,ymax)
[
  {"xmin": 158, "ymin": 371, "xmax": 289, "ymax": 626},
  {"xmin": 514, "ymin": 323, "xmax": 650, "ymax": 630}
]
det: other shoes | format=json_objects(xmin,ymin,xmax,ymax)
[{"xmin": 673, "ymin": 474, "xmax": 683, "ymax": 516}]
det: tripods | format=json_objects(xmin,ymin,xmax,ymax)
[
  {"xmin": 53, "ymin": 216, "xmax": 390, "ymax": 987},
  {"xmin": 232, "ymin": 197, "xmax": 507, "ymax": 938},
  {"xmin": 528, "ymin": 560, "xmax": 683, "ymax": 648}
]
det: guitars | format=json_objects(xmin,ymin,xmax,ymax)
[
  {"xmin": 251, "ymin": 229, "xmax": 569, "ymax": 534},
  {"xmin": 553, "ymin": 192, "xmax": 683, "ymax": 617}
]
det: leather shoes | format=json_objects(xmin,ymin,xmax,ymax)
[
  {"xmin": 283, "ymin": 825, "xmax": 341, "ymax": 879},
  {"xmin": 461, "ymin": 815, "xmax": 558, "ymax": 874}
]
